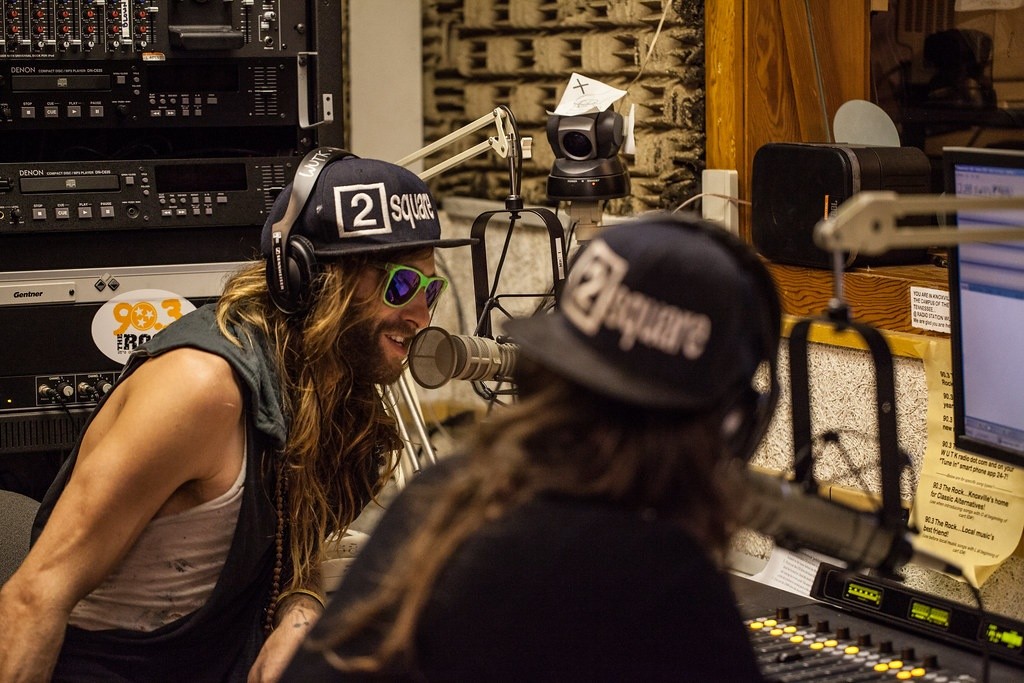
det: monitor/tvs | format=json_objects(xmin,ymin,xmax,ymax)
[{"xmin": 941, "ymin": 144, "xmax": 1024, "ymax": 468}]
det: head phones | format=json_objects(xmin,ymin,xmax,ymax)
[
  {"xmin": 264, "ymin": 147, "xmax": 361, "ymax": 321},
  {"xmin": 637, "ymin": 208, "xmax": 787, "ymax": 470}
]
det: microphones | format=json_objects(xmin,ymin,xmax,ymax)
[
  {"xmin": 408, "ymin": 327, "xmax": 520, "ymax": 389},
  {"xmin": 731, "ymin": 465, "xmax": 963, "ymax": 578}
]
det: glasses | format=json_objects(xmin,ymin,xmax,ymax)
[{"xmin": 365, "ymin": 259, "xmax": 448, "ymax": 313}]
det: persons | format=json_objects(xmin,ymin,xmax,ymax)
[
  {"xmin": 276, "ymin": 211, "xmax": 784, "ymax": 683},
  {"xmin": 0, "ymin": 147, "xmax": 480, "ymax": 683}
]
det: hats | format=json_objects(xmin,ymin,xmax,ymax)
[
  {"xmin": 260, "ymin": 155, "xmax": 480, "ymax": 262},
  {"xmin": 498, "ymin": 211, "xmax": 786, "ymax": 418}
]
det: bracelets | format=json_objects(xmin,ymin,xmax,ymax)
[{"xmin": 276, "ymin": 589, "xmax": 326, "ymax": 610}]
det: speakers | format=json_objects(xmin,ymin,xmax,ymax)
[{"xmin": 749, "ymin": 143, "xmax": 935, "ymax": 272}]
